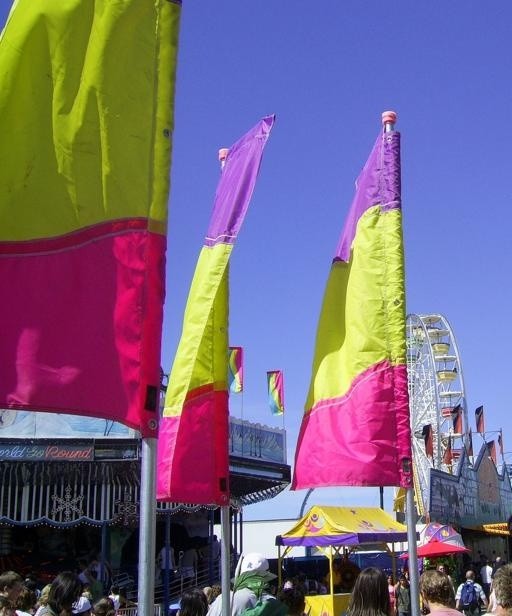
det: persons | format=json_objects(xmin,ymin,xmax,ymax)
[{"xmin": 1, "ymin": 533, "xmax": 511, "ymax": 616}]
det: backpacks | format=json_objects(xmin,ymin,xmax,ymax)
[{"xmin": 460, "ymin": 582, "xmax": 479, "ymax": 610}]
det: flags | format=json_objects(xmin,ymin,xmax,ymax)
[
  {"xmin": 267, "ymin": 370, "xmax": 286, "ymax": 416},
  {"xmin": 467, "ymin": 428, "xmax": 473, "ymax": 458},
  {"xmin": 474, "ymin": 405, "xmax": 484, "ymax": 434},
  {"xmin": 452, "ymin": 404, "xmax": 462, "ymax": 436},
  {"xmin": 290, "ymin": 126, "xmax": 415, "ymax": 496},
  {"xmin": 227, "ymin": 344, "xmax": 243, "ymax": 393},
  {"xmin": 487, "ymin": 440, "xmax": 497, "ymax": 463},
  {"xmin": 441, "ymin": 434, "xmax": 453, "ymax": 465},
  {"xmin": 421, "ymin": 424, "xmax": 432, "ymax": 458},
  {"xmin": 0, "ymin": 0, "xmax": 174, "ymax": 446},
  {"xmin": 497, "ymin": 427, "xmax": 504, "ymax": 454},
  {"xmin": 154, "ymin": 113, "xmax": 277, "ymax": 506}
]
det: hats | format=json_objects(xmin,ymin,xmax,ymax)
[
  {"xmin": 72, "ymin": 597, "xmax": 91, "ymax": 614},
  {"xmin": 230, "ymin": 555, "xmax": 277, "ymax": 584}
]
